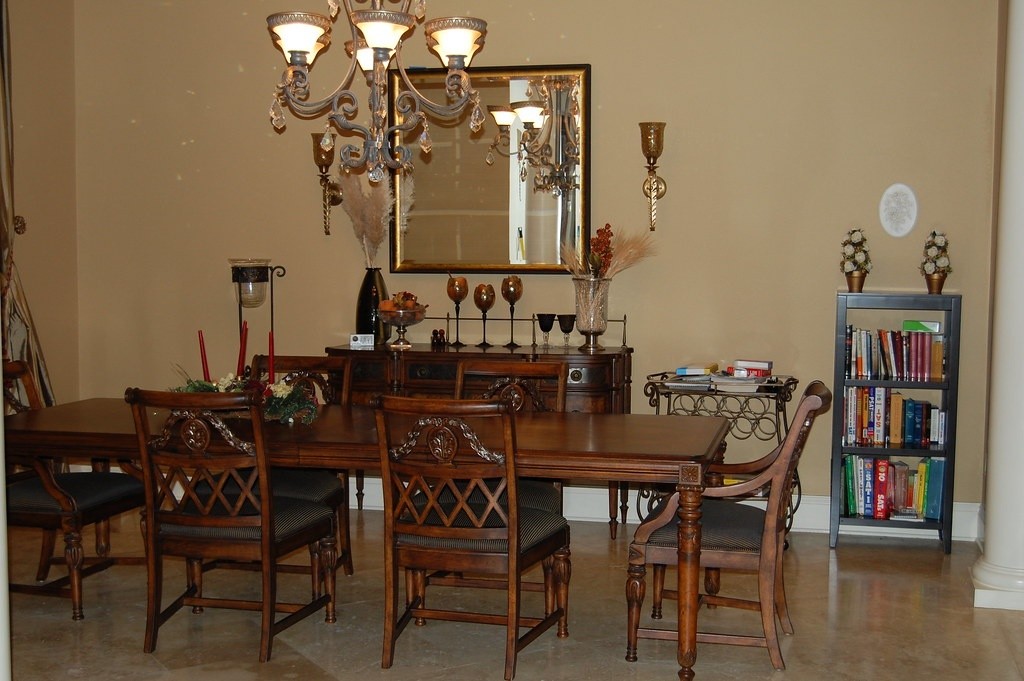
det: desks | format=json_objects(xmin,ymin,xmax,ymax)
[
  {"xmin": 326, "ymin": 343, "xmax": 630, "ymax": 539},
  {"xmin": 6, "ymin": 397, "xmax": 737, "ymax": 680}
]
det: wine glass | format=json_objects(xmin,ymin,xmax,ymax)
[
  {"xmin": 536, "ymin": 313, "xmax": 555, "ymax": 349},
  {"xmin": 474, "ymin": 285, "xmax": 496, "ymax": 347},
  {"xmin": 376, "ymin": 307, "xmax": 426, "ymax": 350},
  {"xmin": 501, "ymin": 279, "xmax": 524, "ymax": 347},
  {"xmin": 557, "ymin": 314, "xmax": 576, "ymax": 348},
  {"xmin": 447, "ymin": 278, "xmax": 468, "ymax": 347}
]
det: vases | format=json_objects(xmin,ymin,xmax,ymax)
[
  {"xmin": 356, "ymin": 268, "xmax": 394, "ymax": 344},
  {"xmin": 846, "ymin": 268, "xmax": 866, "ymax": 292},
  {"xmin": 929, "ymin": 272, "xmax": 944, "ymax": 294},
  {"xmin": 572, "ymin": 280, "xmax": 614, "ymax": 350}
]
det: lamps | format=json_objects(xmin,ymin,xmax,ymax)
[
  {"xmin": 484, "ymin": 74, "xmax": 584, "ymax": 199},
  {"xmin": 267, "ymin": 0, "xmax": 488, "ymax": 235},
  {"xmin": 637, "ymin": 121, "xmax": 668, "ymax": 229},
  {"xmin": 306, "ymin": 133, "xmax": 344, "ymax": 233}
]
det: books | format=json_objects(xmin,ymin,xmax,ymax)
[
  {"xmin": 842, "ymin": 385, "xmax": 945, "ymax": 447},
  {"xmin": 666, "ymin": 360, "xmax": 773, "ymax": 392},
  {"xmin": 840, "ymin": 453, "xmax": 944, "ymax": 522},
  {"xmin": 846, "ymin": 325, "xmax": 946, "ymax": 379}
]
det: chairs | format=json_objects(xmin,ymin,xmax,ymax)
[
  {"xmin": 372, "ymin": 406, "xmax": 575, "ymax": 681},
  {"xmin": 127, "ymin": 388, "xmax": 339, "ymax": 664},
  {"xmin": 5, "ymin": 356, "xmax": 157, "ymax": 619},
  {"xmin": 621, "ymin": 378, "xmax": 834, "ymax": 671},
  {"xmin": 405, "ymin": 360, "xmax": 577, "ymax": 594},
  {"xmin": 205, "ymin": 352, "xmax": 358, "ymax": 578}
]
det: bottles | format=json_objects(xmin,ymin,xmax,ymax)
[
  {"xmin": 431, "ymin": 329, "xmax": 438, "ymax": 345},
  {"xmin": 438, "ymin": 329, "xmax": 445, "ymax": 345},
  {"xmin": 227, "ymin": 257, "xmax": 271, "ymax": 308}
]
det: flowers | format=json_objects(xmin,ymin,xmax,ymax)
[
  {"xmin": 558, "ymin": 226, "xmax": 653, "ymax": 283},
  {"xmin": 919, "ymin": 233, "xmax": 954, "ymax": 273},
  {"xmin": 838, "ymin": 228, "xmax": 872, "ymax": 273}
]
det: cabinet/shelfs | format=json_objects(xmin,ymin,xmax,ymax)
[
  {"xmin": 635, "ymin": 376, "xmax": 803, "ymax": 549},
  {"xmin": 829, "ymin": 292, "xmax": 962, "ymax": 547}
]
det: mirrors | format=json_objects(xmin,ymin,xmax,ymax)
[{"xmin": 387, "ymin": 63, "xmax": 592, "ymax": 274}]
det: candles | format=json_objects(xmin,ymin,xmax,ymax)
[
  {"xmin": 270, "ymin": 332, "xmax": 276, "ymax": 386},
  {"xmin": 196, "ymin": 331, "xmax": 209, "ymax": 379},
  {"xmin": 237, "ymin": 320, "xmax": 249, "ymax": 377}
]
